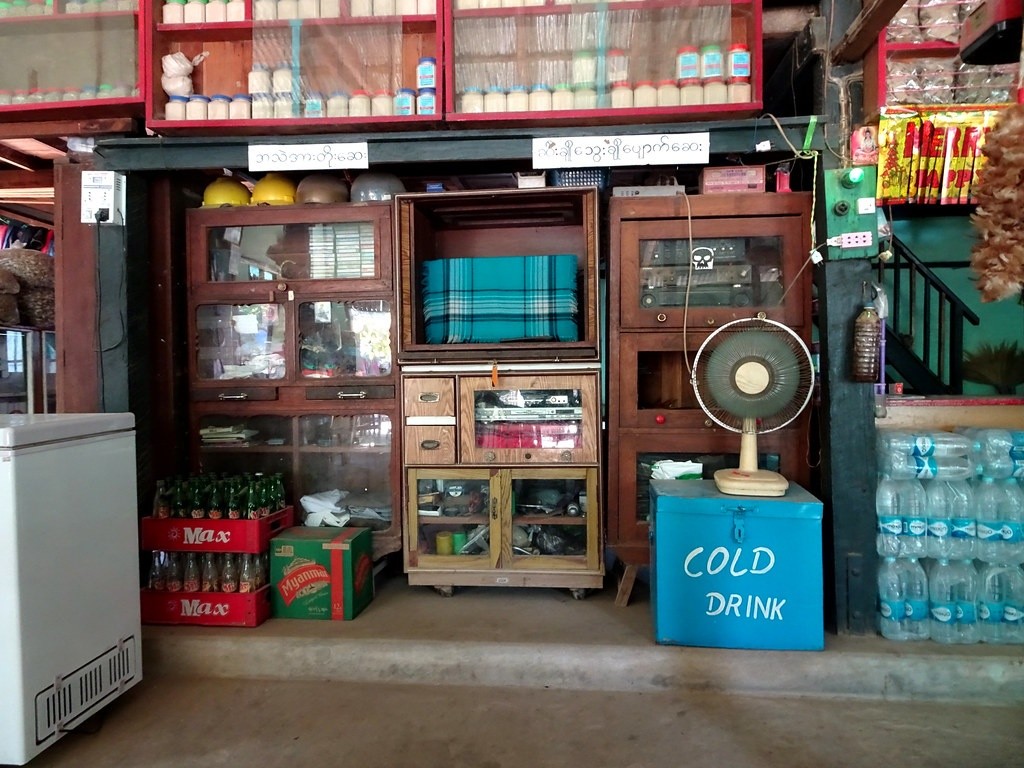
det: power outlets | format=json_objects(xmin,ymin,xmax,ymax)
[
  {"xmin": 857, "ymin": 198, "xmax": 876, "ymax": 214},
  {"xmin": 80, "ymin": 170, "xmax": 127, "ymax": 228},
  {"xmin": 839, "ymin": 231, "xmax": 872, "ymax": 249}
]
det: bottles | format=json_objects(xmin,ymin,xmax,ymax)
[
  {"xmin": 0, "ymin": 0, "xmax": 752, "ymax": 120},
  {"xmin": 875, "ymin": 425, "xmax": 1024, "ymax": 644},
  {"xmin": 851, "ymin": 302, "xmax": 881, "ymax": 382},
  {"xmin": 148, "ymin": 471, "xmax": 287, "ymax": 594}
]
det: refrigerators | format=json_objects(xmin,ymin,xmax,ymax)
[{"xmin": 0, "ymin": 413, "xmax": 143, "ymax": 765}]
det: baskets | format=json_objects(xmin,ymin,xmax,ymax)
[{"xmin": 551, "ymin": 167, "xmax": 605, "ymax": 192}]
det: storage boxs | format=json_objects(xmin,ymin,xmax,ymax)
[
  {"xmin": 551, "ymin": 167, "xmax": 605, "ymax": 190},
  {"xmin": 270, "ymin": 525, "xmax": 375, "ymax": 621},
  {"xmin": 649, "ymin": 478, "xmax": 825, "ymax": 652},
  {"xmin": 140, "ymin": 585, "xmax": 270, "ymax": 626},
  {"xmin": 140, "ymin": 505, "xmax": 294, "ymax": 553},
  {"xmin": 701, "ymin": 165, "xmax": 767, "ymax": 194}
]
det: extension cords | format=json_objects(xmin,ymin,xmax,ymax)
[{"xmin": 838, "ymin": 232, "xmax": 872, "ymax": 250}]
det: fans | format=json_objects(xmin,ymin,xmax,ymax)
[{"xmin": 690, "ymin": 318, "xmax": 815, "ymax": 496}]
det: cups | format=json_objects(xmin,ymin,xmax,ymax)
[
  {"xmin": 451, "ymin": 531, "xmax": 467, "ymax": 555},
  {"xmin": 436, "ymin": 531, "xmax": 452, "ymax": 555}
]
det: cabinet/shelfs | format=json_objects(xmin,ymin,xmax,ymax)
[
  {"xmin": 609, "ymin": 192, "xmax": 812, "ymax": 606},
  {"xmin": 1, "ymin": 0, "xmax": 145, "ymax": 118},
  {"xmin": 143, "ymin": 0, "xmax": 444, "ymax": 136},
  {"xmin": 185, "ymin": 205, "xmax": 404, "ymax": 562},
  {"xmin": 444, "ymin": 0, "xmax": 764, "ymax": 128},
  {"xmin": 396, "ymin": 186, "xmax": 605, "ymax": 599},
  {"xmin": 866, "ymin": 1, "xmax": 1024, "ymax": 122}
]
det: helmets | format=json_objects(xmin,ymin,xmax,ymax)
[
  {"xmin": 351, "ymin": 170, "xmax": 406, "ymax": 201},
  {"xmin": 250, "ymin": 173, "xmax": 296, "ymax": 205},
  {"xmin": 201, "ymin": 177, "xmax": 251, "ymax": 209},
  {"xmin": 295, "ymin": 174, "xmax": 348, "ymax": 204}
]
